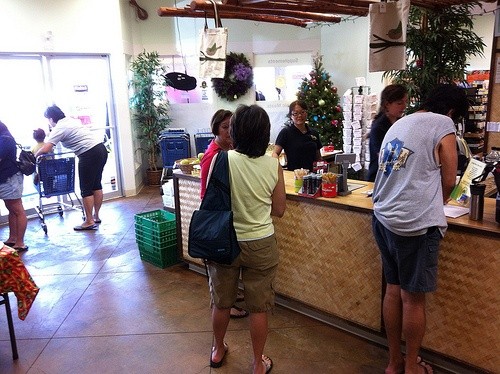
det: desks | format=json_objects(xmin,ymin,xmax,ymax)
[{"xmin": 0, "ymin": 245, "xmax": 40, "ymax": 360}]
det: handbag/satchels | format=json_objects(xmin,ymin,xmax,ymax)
[{"xmin": 188, "ymin": 209, "xmax": 241, "ymax": 264}]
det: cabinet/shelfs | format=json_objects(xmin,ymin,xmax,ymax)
[{"xmin": 463, "ymin": 87, "xmax": 488, "ymax": 158}]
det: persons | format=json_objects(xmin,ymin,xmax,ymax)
[
  {"xmin": 272, "ymin": 101, "xmax": 323, "ymax": 172},
  {"xmin": 203, "ymin": 109, "xmax": 254, "ymax": 318},
  {"xmin": 0, "ymin": 120, "xmax": 28, "ymax": 253},
  {"xmin": 31, "ymin": 128, "xmax": 56, "ymax": 198},
  {"xmin": 206, "ymin": 104, "xmax": 289, "ymax": 374},
  {"xmin": 34, "ymin": 103, "xmax": 108, "ymax": 231},
  {"xmin": 365, "ymin": 85, "xmax": 410, "ymax": 182},
  {"xmin": 366, "ymin": 84, "xmax": 470, "ymax": 374}
]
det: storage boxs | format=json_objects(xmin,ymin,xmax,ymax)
[{"xmin": 134, "ymin": 209, "xmax": 178, "ymax": 269}]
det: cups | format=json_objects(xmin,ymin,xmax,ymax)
[{"xmin": 294, "ymin": 178, "xmax": 302, "ymax": 193}]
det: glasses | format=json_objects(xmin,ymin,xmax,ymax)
[{"xmin": 291, "ymin": 111, "xmax": 307, "ymax": 116}]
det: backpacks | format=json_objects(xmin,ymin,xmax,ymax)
[{"xmin": 0, "ymin": 135, "xmax": 36, "ymax": 176}]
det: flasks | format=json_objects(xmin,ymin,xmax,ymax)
[{"xmin": 468, "ymin": 184, "xmax": 486, "ymax": 221}]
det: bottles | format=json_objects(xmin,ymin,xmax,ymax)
[{"xmin": 302, "ymin": 173, "xmax": 322, "ymax": 194}]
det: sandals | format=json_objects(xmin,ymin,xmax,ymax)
[
  {"xmin": 73, "ymin": 223, "xmax": 98, "ymax": 230},
  {"xmin": 82, "ymin": 216, "xmax": 101, "ymax": 223}
]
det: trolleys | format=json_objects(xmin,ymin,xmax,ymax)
[
  {"xmin": 156, "ymin": 123, "xmax": 217, "ymax": 194},
  {"xmin": 33, "ymin": 151, "xmax": 87, "ymax": 235}
]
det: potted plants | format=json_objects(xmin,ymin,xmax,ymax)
[{"xmin": 127, "ymin": 48, "xmax": 176, "ymax": 184}]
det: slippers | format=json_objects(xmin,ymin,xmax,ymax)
[
  {"xmin": 210, "ymin": 343, "xmax": 228, "ymax": 368},
  {"xmin": 3, "ymin": 242, "xmax": 28, "ymax": 252},
  {"xmin": 384, "ymin": 355, "xmax": 435, "ymax": 374},
  {"xmin": 230, "ymin": 305, "xmax": 248, "ymax": 318},
  {"xmin": 262, "ymin": 356, "xmax": 273, "ymax": 374},
  {"xmin": 236, "ymin": 293, "xmax": 244, "ymax": 302}
]
